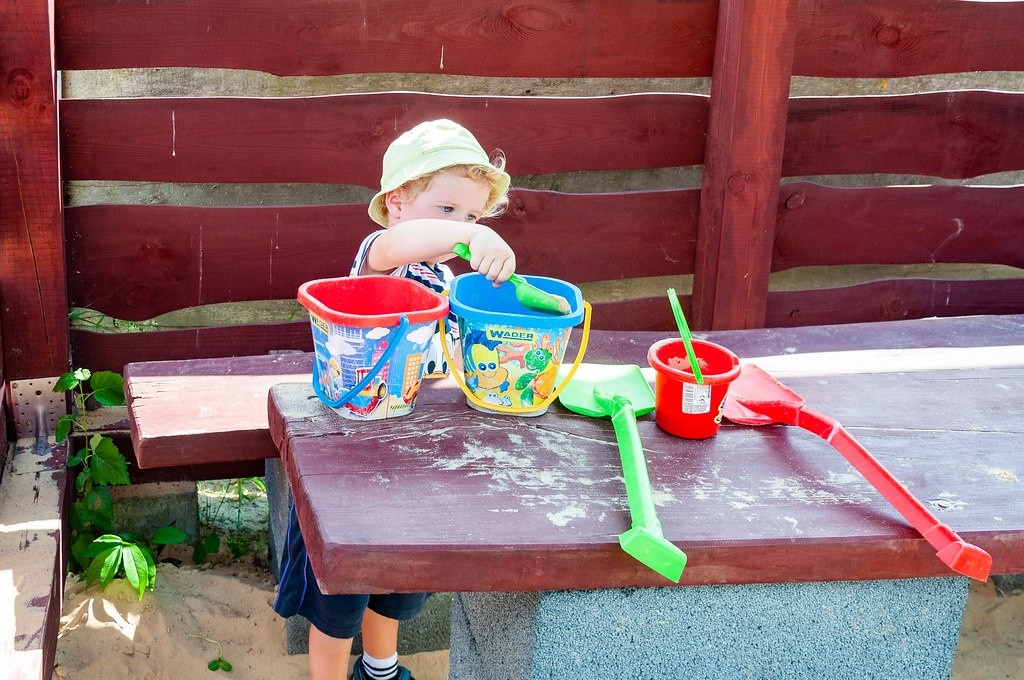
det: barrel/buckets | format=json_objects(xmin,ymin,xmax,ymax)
[
  {"xmin": 439, "ymin": 271, "xmax": 593, "ymax": 417},
  {"xmin": 646, "ymin": 338, "xmax": 741, "ymax": 440},
  {"xmin": 296, "ymin": 274, "xmax": 450, "ymax": 421}
]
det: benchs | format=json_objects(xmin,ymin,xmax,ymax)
[{"xmin": 124, "ymin": 314, "xmax": 1023, "ymax": 469}]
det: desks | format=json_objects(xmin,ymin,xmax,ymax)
[{"xmin": 268, "ymin": 365, "xmax": 1024, "ymax": 680}]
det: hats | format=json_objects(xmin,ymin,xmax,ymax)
[{"xmin": 368, "ymin": 119, "xmax": 511, "ymax": 229}]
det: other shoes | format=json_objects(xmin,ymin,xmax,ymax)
[{"xmin": 348, "ymin": 653, "xmax": 416, "ymax": 680}]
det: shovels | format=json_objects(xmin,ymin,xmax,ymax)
[
  {"xmin": 553, "ymin": 363, "xmax": 689, "ymax": 583},
  {"xmin": 723, "ymin": 362, "xmax": 994, "ymax": 583},
  {"xmin": 450, "ymin": 242, "xmax": 570, "ymax": 316}
]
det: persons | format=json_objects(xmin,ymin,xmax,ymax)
[{"xmin": 273, "ymin": 120, "xmax": 517, "ymax": 680}]
